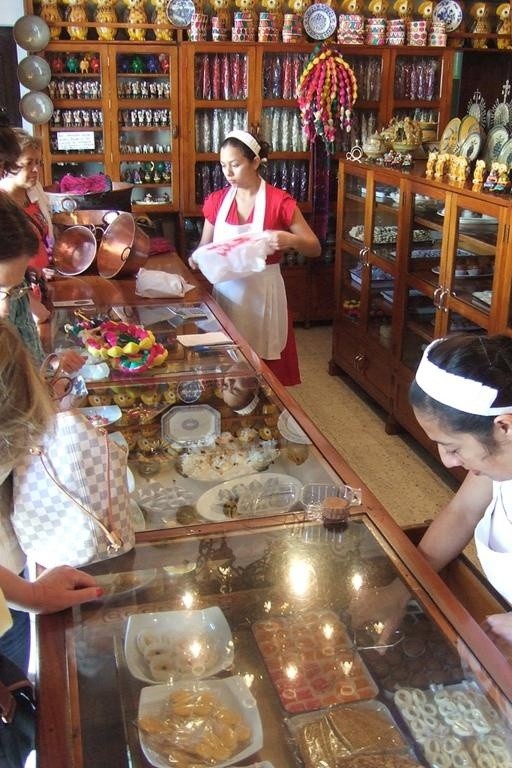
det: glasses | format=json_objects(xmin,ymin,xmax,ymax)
[{"xmin": 0, "ymin": 279, "xmax": 30, "ymax": 301}]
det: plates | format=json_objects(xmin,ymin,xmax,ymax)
[
  {"xmin": 439, "ymin": 114, "xmax": 511, "ymax": 173},
  {"xmin": 84, "ymin": 569, "xmax": 159, "ymax": 607},
  {"xmin": 160, "ymin": 404, "xmax": 225, "ymax": 452},
  {"xmin": 275, "ymin": 408, "xmax": 316, "ymax": 450},
  {"xmin": 164, "ymin": 0, "xmax": 197, "ymax": 28},
  {"xmin": 194, "ymin": 469, "xmax": 304, "ymax": 526},
  {"xmin": 303, "ymin": 3, "xmax": 337, "ymax": 40},
  {"xmin": 433, "ymin": 0, "xmax": 464, "ymax": 33},
  {"xmin": 76, "ymin": 402, "xmax": 123, "ymax": 429},
  {"xmin": 121, "ymin": 604, "xmax": 238, "ymax": 687},
  {"xmin": 136, "ymin": 670, "xmax": 263, "ymax": 767},
  {"xmin": 13, "ymin": 12, "xmax": 53, "ymax": 53}
]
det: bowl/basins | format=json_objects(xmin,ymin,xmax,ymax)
[
  {"xmin": 18, "ymin": 89, "xmax": 55, "ymax": 127},
  {"xmin": 40, "ymin": 178, "xmax": 136, "ymax": 214},
  {"xmin": 52, "ymin": 221, "xmax": 98, "ymax": 280},
  {"xmin": 49, "ymin": 207, "xmax": 132, "ymax": 241}
]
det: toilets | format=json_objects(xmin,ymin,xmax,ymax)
[{"xmin": 53, "ymin": 223, "xmax": 104, "ymax": 274}]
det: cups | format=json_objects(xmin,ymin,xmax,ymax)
[{"xmin": 431, "ymin": 260, "xmax": 494, "ymax": 279}]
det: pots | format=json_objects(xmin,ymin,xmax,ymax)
[{"xmin": 18, "ymin": 56, "xmax": 54, "ymax": 91}]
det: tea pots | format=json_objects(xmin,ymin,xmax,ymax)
[{"xmin": 362, "ymin": 130, "xmax": 390, "ymax": 161}]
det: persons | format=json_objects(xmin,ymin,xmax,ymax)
[
  {"xmin": 0, "ymin": 111, "xmax": 91, "ymax": 390},
  {"xmin": 345, "ymin": 332, "xmax": 512, "ymax": 657},
  {"xmin": 187, "ymin": 130, "xmax": 325, "ymax": 388},
  {"xmin": 221, "ymin": 361, "xmax": 259, "ymax": 415},
  {"xmin": 0, "ymin": 313, "xmax": 103, "ymax": 767}
]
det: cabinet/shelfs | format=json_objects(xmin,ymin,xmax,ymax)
[
  {"xmin": 309, "ymin": 44, "xmax": 454, "ymax": 325},
  {"xmin": 36, "ymin": 39, "xmax": 179, "ymax": 216},
  {"xmin": 184, "ymin": 44, "xmax": 315, "ymax": 328},
  {"xmin": 328, "ymin": 154, "xmax": 512, "ymax": 484},
  {"xmin": 36, "ymin": 250, "xmax": 327, "ymax": 482}
]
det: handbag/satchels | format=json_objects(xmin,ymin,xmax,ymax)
[
  {"xmin": 0, "ymin": 656, "xmax": 40, "ymax": 768},
  {"xmin": 9, "ymin": 354, "xmax": 136, "ymax": 570}
]
